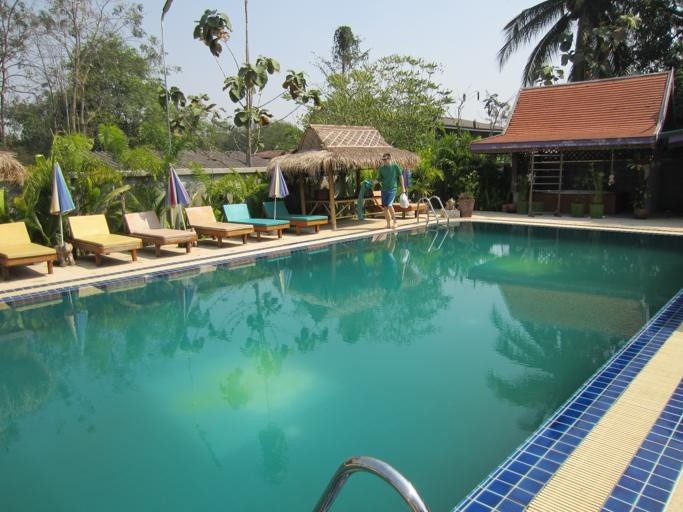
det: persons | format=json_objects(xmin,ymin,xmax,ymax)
[
  {"xmin": 371, "ymin": 153, "xmax": 407, "ymax": 229},
  {"xmin": 371, "ymin": 233, "xmax": 407, "ymax": 300}
]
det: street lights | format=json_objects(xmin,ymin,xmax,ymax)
[{"xmin": 161, "ymin": 0, "xmax": 174, "ymax": 156}]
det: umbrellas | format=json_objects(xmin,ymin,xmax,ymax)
[
  {"xmin": 175, "ymin": 277, "xmax": 199, "ymax": 324},
  {"xmin": 275, "ymin": 260, "xmax": 294, "ymax": 304},
  {"xmin": 61, "ymin": 291, "xmax": 89, "ymax": 359},
  {"xmin": 49, "ymin": 158, "xmax": 75, "ymax": 244},
  {"xmin": 268, "ymin": 160, "xmax": 291, "ymax": 220},
  {"xmin": 164, "ymin": 160, "xmax": 193, "ymax": 230}
]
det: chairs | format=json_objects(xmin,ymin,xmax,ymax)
[
  {"xmin": 184, "ymin": 205, "xmax": 254, "ymax": 247},
  {"xmin": 68, "ymin": 214, "xmax": 144, "ymax": 267},
  {"xmin": 223, "ymin": 203, "xmax": 291, "ymax": 239},
  {"xmin": 1, "ymin": 221, "xmax": 57, "ymax": 281},
  {"xmin": 121, "ymin": 210, "xmax": 199, "ymax": 257},
  {"xmin": 373, "ymin": 190, "xmax": 430, "ymax": 219},
  {"xmin": 263, "ymin": 200, "xmax": 329, "ymax": 234}
]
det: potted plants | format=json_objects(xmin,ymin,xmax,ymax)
[
  {"xmin": 444, "ymin": 191, "xmax": 476, "ymax": 219},
  {"xmin": 631, "ymin": 185, "xmax": 652, "ymax": 219},
  {"xmin": 569, "ymin": 163, "xmax": 606, "ymax": 219},
  {"xmin": 502, "ymin": 174, "xmax": 545, "ymax": 215}
]
